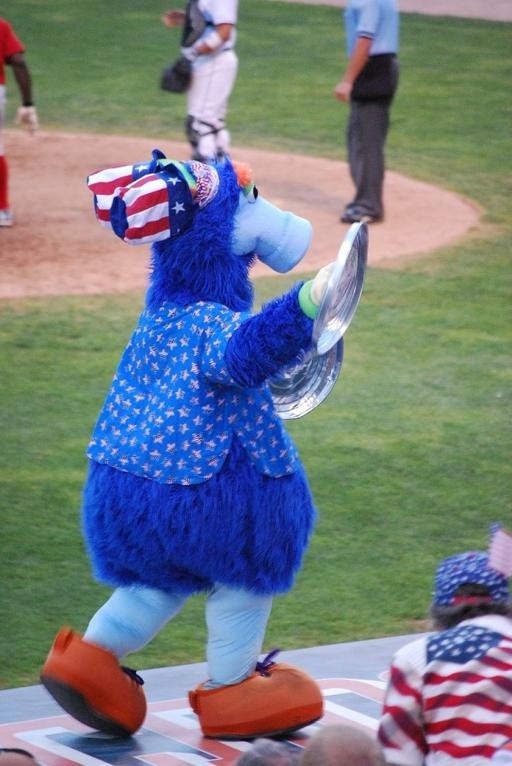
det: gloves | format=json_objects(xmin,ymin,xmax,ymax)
[{"xmin": 13, "ymin": 105, "xmax": 40, "ymax": 136}]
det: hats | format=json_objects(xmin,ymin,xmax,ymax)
[{"xmin": 431, "ymin": 548, "xmax": 511, "ymax": 613}]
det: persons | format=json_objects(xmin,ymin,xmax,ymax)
[
  {"xmin": 230, "ymin": 724, "xmax": 387, "ymax": 766},
  {"xmin": 0, "ymin": 17, "xmax": 38, "ymax": 226},
  {"xmin": 159, "ymin": 0, "xmax": 239, "ymax": 159},
  {"xmin": 0, "ymin": 747, "xmax": 39, "ymax": 766},
  {"xmin": 377, "ymin": 550, "xmax": 512, "ymax": 766},
  {"xmin": 334, "ymin": 0, "xmax": 400, "ymax": 224}
]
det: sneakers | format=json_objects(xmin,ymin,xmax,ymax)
[
  {"xmin": 0, "ymin": 209, "xmax": 13, "ymax": 227},
  {"xmin": 339, "ymin": 206, "xmax": 381, "ymax": 224}
]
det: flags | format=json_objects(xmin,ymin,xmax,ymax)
[{"xmin": 484, "ymin": 520, "xmax": 512, "ymax": 579}]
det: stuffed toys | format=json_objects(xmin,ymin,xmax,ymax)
[{"xmin": 42, "ymin": 149, "xmax": 369, "ymax": 740}]
